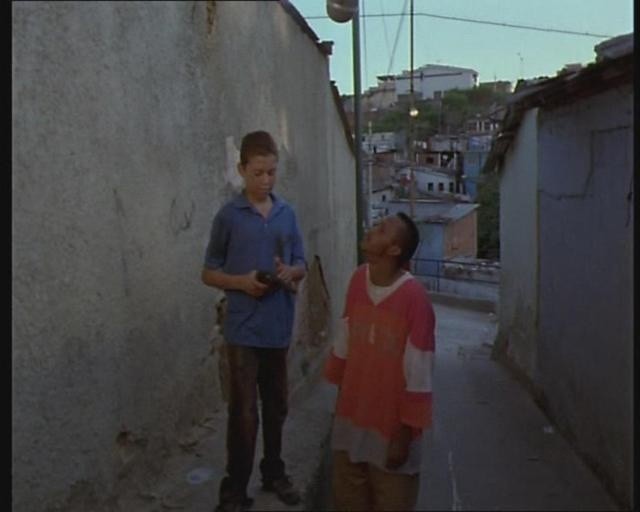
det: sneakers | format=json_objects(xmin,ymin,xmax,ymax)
[{"xmin": 261, "ymin": 475, "xmax": 300, "ymax": 505}]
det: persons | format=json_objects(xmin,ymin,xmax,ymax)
[
  {"xmin": 202, "ymin": 130, "xmax": 309, "ymax": 509},
  {"xmin": 323, "ymin": 211, "xmax": 436, "ymax": 512}
]
